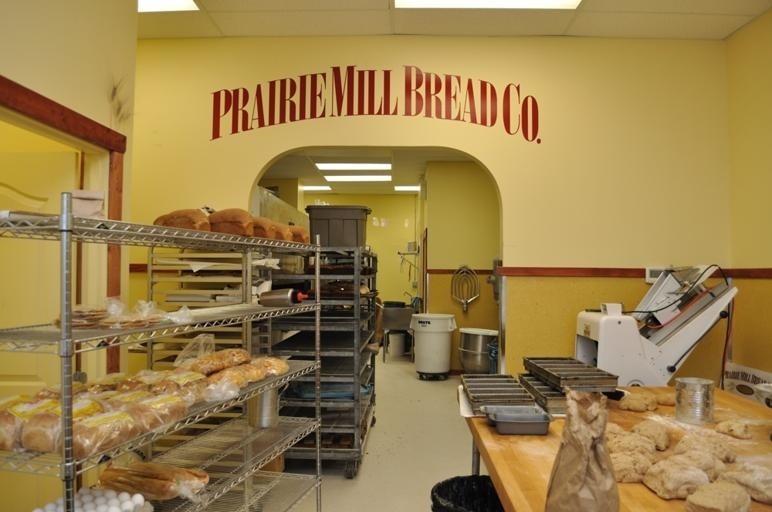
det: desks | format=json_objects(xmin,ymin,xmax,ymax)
[{"xmin": 458, "ymin": 387, "xmax": 770, "ymax": 511}]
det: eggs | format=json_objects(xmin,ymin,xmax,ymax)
[{"xmin": 32, "ymin": 487, "xmax": 145, "ymax": 512}]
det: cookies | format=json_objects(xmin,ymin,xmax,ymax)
[{"xmin": 55, "ymin": 306, "xmax": 164, "ymax": 328}]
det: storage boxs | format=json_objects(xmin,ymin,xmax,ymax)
[{"xmin": 306, "ymin": 205, "xmax": 373, "ymax": 248}]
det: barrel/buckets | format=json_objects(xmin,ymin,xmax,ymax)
[{"xmin": 389, "ymin": 333, "xmax": 405, "ymax": 356}]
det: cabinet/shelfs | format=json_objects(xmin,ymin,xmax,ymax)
[{"xmin": 4, "ymin": 210, "xmax": 376, "ymax": 511}]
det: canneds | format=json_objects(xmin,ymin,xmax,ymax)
[{"xmin": 674, "ymin": 376, "xmax": 717, "ymax": 424}]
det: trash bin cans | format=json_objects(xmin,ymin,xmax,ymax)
[{"xmin": 430, "ymin": 473, "xmax": 505, "ymax": 512}]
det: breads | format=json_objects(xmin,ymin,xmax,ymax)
[
  {"xmin": 0, "ymin": 370, "xmax": 205, "ymax": 461},
  {"xmin": 152, "ymin": 208, "xmax": 310, "ymax": 244},
  {"xmin": 98, "ymin": 461, "xmax": 208, "ymax": 500},
  {"xmin": 308, "ymin": 280, "xmax": 370, "ymax": 312},
  {"xmin": 183, "ymin": 349, "xmax": 288, "ymax": 391}
]
